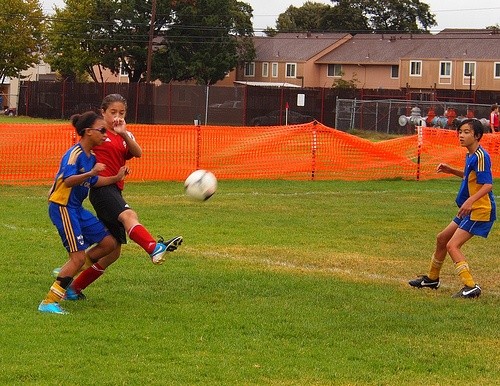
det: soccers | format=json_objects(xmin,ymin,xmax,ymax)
[{"xmin": 183, "ymin": 170, "xmax": 217, "ymax": 203}]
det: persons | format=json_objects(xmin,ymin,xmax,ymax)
[
  {"xmin": 408, "ymin": 119, "xmax": 496, "ymax": 298},
  {"xmin": 445, "ymin": 107, "xmax": 456, "ymax": 125},
  {"xmin": 38, "ymin": 111, "xmax": 126, "ymax": 315},
  {"xmin": 63, "ymin": 94, "xmax": 183, "ymax": 301},
  {"xmin": 466, "ymin": 108, "xmax": 474, "ymax": 120},
  {"xmin": 490, "ymin": 104, "xmax": 500, "ymax": 133},
  {"xmin": 427, "ymin": 107, "xmax": 435, "ymax": 124}
]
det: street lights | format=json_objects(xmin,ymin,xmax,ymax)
[
  {"xmin": 296, "ymin": 75, "xmax": 304, "ymax": 93},
  {"xmin": 464, "ymin": 71, "xmax": 472, "ymax": 91}
]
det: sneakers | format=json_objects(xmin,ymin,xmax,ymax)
[
  {"xmin": 52, "ymin": 268, "xmax": 62, "ymax": 279},
  {"xmin": 150, "ymin": 234, "xmax": 183, "ymax": 265},
  {"xmin": 407, "ymin": 274, "xmax": 439, "ymax": 291},
  {"xmin": 38, "ymin": 300, "xmax": 70, "ymax": 316},
  {"xmin": 451, "ymin": 284, "xmax": 481, "ymax": 299},
  {"xmin": 62, "ymin": 288, "xmax": 86, "ymax": 301}
]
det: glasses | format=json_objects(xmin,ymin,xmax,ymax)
[{"xmin": 84, "ymin": 126, "xmax": 106, "ymax": 134}]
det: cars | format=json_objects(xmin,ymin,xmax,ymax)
[
  {"xmin": 5, "ymin": 102, "xmax": 59, "ymax": 118},
  {"xmin": 208, "ymin": 101, "xmax": 242, "ymax": 109},
  {"xmin": 249, "ymin": 109, "xmax": 313, "ymax": 127}
]
